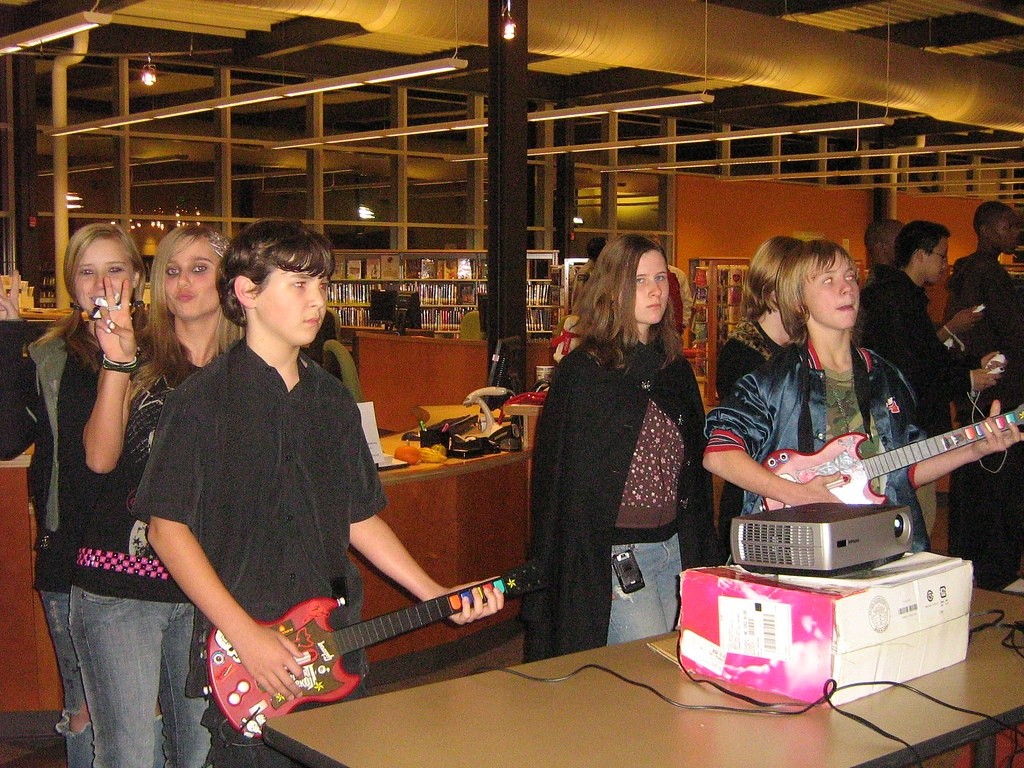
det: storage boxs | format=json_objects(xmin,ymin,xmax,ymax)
[{"xmin": 648, "ymin": 552, "xmax": 974, "ymax": 709}]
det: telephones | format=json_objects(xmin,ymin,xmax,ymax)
[{"xmin": 505, "ymin": 388, "xmax": 548, "ymax": 404}]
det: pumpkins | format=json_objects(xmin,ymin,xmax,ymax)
[{"xmin": 395, "ymin": 440, "xmax": 419, "ymax": 464}]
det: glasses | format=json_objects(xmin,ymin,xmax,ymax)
[{"xmin": 913, "ymin": 248, "xmax": 948, "ymax": 264}]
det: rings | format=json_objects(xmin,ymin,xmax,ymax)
[{"xmin": 106, "ymin": 305, "xmax": 119, "ymax": 312}]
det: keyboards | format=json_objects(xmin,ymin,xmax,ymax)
[{"xmin": 427, "ymin": 414, "xmax": 478, "ymax": 437}]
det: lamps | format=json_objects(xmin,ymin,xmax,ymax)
[{"xmin": 0, "ymin": 0, "xmax": 1024, "ymax": 205}]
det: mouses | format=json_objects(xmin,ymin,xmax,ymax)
[{"xmin": 402, "ymin": 432, "xmax": 421, "ymax": 441}]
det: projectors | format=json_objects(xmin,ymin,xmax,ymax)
[{"xmin": 730, "ymin": 502, "xmax": 915, "ymax": 578}]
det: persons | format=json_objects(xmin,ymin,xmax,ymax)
[
  {"xmin": 572, "ymin": 234, "xmax": 695, "ymax": 349},
  {"xmin": 134, "ymin": 217, "xmax": 504, "ymax": 768},
  {"xmin": 702, "ymin": 200, "xmax": 1024, "ymax": 593},
  {"xmin": 522, "ymin": 232, "xmax": 713, "ymax": 665},
  {"xmin": 0, "ymin": 222, "xmax": 244, "ymax": 768}
]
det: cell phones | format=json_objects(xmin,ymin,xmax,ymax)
[{"xmin": 611, "ymin": 550, "xmax": 645, "ymax": 594}]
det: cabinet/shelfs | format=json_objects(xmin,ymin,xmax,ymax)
[
  {"xmin": 328, "ymin": 248, "xmax": 590, "ymax": 336},
  {"xmin": 688, "ymin": 261, "xmax": 743, "ymax": 407},
  {"xmin": 39, "ymin": 267, "xmax": 56, "ymax": 290}
]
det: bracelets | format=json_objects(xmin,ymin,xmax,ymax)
[{"xmin": 102, "ymin": 354, "xmax": 138, "ymax": 373}]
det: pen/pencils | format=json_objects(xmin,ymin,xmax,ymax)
[
  {"xmin": 419, "ymin": 420, "xmax": 426, "ymax": 432},
  {"xmin": 440, "ymin": 423, "xmax": 450, "ymax": 432}
]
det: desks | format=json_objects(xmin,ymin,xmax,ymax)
[{"xmin": 263, "ymin": 586, "xmax": 1024, "ymax": 768}]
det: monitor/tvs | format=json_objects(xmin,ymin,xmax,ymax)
[
  {"xmin": 369, "ymin": 290, "xmax": 398, "ymax": 331},
  {"xmin": 480, "ymin": 336, "xmax": 524, "ymax": 424},
  {"xmin": 394, "ymin": 291, "xmax": 422, "ymax": 336}
]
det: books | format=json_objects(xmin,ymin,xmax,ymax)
[
  {"xmin": 526, "ymin": 284, "xmax": 553, "ymax": 331},
  {"xmin": 330, "ymin": 281, "xmax": 485, "ymax": 305},
  {"xmin": 337, "ymin": 306, "xmax": 478, "ymax": 330}
]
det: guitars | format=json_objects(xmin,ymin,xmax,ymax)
[
  {"xmin": 759, "ymin": 403, "xmax": 1024, "ymax": 516},
  {"xmin": 194, "ymin": 562, "xmax": 551, "ymax": 735}
]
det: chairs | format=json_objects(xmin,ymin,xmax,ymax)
[
  {"xmin": 459, "ymin": 310, "xmax": 486, "ymax": 339},
  {"xmin": 322, "ymin": 341, "xmax": 365, "ymax": 403}
]
